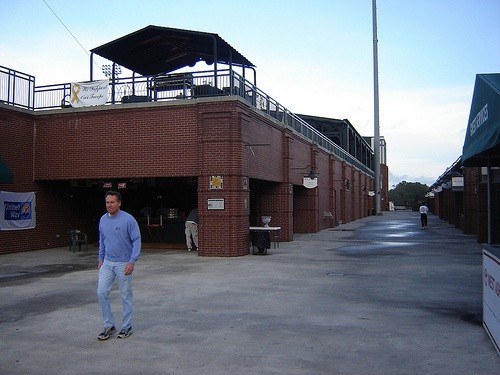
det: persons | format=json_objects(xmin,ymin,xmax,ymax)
[
  {"xmin": 98, "ymin": 191, "xmax": 141, "ymax": 340},
  {"xmin": 185, "ymin": 208, "xmax": 198, "ymax": 251},
  {"xmin": 420, "ymin": 201, "xmax": 429, "ymax": 229}
]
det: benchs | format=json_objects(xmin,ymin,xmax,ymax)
[{"xmin": 146, "ymin": 72, "xmax": 193, "ymax": 101}]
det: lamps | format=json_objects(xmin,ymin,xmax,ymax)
[
  {"xmin": 291, "ymin": 165, "xmax": 318, "ymax": 180},
  {"xmin": 360, "ymin": 184, "xmax": 381, "ymax": 196},
  {"xmin": 334, "ymin": 178, "xmax": 352, "ymax": 192}
]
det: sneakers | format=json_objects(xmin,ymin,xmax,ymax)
[
  {"xmin": 118, "ymin": 326, "xmax": 134, "ymax": 339},
  {"xmin": 97, "ymin": 326, "xmax": 117, "ymax": 340}
]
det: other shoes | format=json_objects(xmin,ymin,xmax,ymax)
[
  {"xmin": 187, "ymin": 249, "xmax": 191, "ymax": 252},
  {"xmin": 421, "ymin": 226, "xmax": 427, "ymax": 229}
]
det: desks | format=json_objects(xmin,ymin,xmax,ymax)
[{"xmin": 250, "ymin": 227, "xmax": 281, "ymax": 249}]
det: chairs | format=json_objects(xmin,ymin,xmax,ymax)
[
  {"xmin": 252, "ymin": 231, "xmax": 271, "ymax": 255},
  {"xmin": 67, "ymin": 228, "xmax": 88, "ymax": 252}
]
